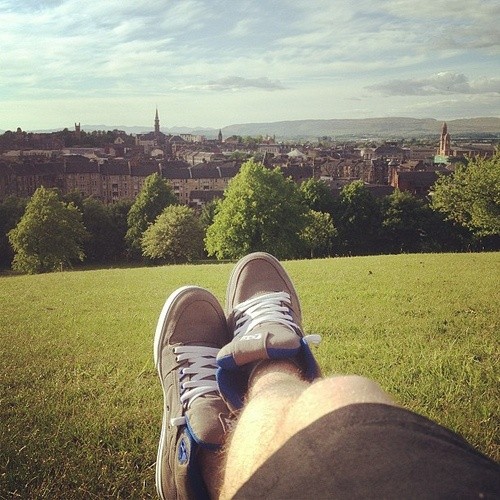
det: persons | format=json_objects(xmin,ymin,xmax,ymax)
[{"xmin": 152, "ymin": 251, "xmax": 500, "ymax": 500}]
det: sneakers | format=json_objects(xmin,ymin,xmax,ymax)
[
  {"xmin": 153, "ymin": 285, "xmax": 231, "ymax": 500},
  {"xmin": 215, "ymin": 250, "xmax": 322, "ymax": 419}
]
